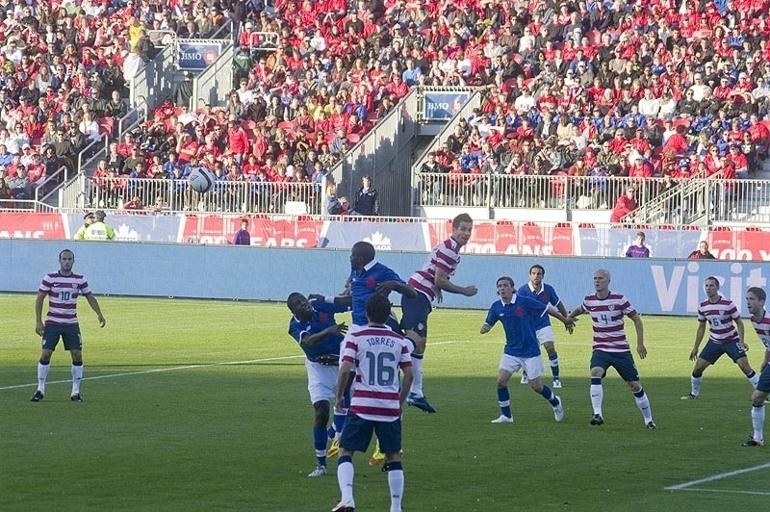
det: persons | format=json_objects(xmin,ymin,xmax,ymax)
[
  {"xmin": 399, "ymin": 214, "xmax": 479, "ymax": 414},
  {"xmin": 480, "ymin": 276, "xmax": 580, "ymax": 424},
  {"xmin": 286, "ymin": 292, "xmax": 356, "ymax": 478},
  {"xmin": 30, "ymin": 249, "xmax": 108, "ymax": 403},
  {"xmin": 680, "ymin": 276, "xmax": 769, "ymax": 406},
  {"xmin": 1, "ymin": 0, "xmax": 770, "ymax": 230},
  {"xmin": 75, "ymin": 210, "xmax": 115, "ymax": 242},
  {"xmin": 520, "ymin": 264, "xmax": 569, "ymax": 389},
  {"xmin": 741, "ymin": 287, "xmax": 770, "ymax": 447},
  {"xmin": 689, "ymin": 241, "xmax": 714, "ymax": 259},
  {"xmin": 570, "ymin": 269, "xmax": 658, "ymax": 431},
  {"xmin": 233, "ymin": 219, "xmax": 250, "ymax": 246},
  {"xmin": 331, "ymin": 296, "xmax": 415, "ymax": 512},
  {"xmin": 309, "ymin": 241, "xmax": 419, "ymax": 465},
  {"xmin": 625, "ymin": 231, "xmax": 649, "ymax": 256}
]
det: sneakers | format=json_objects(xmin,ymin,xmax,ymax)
[
  {"xmin": 520, "ymin": 372, "xmax": 529, "ymax": 384},
  {"xmin": 552, "ymin": 396, "xmax": 564, "ymax": 422},
  {"xmin": 742, "ymin": 435, "xmax": 764, "ymax": 447},
  {"xmin": 31, "ymin": 390, "xmax": 43, "ymax": 401},
  {"xmin": 647, "ymin": 420, "xmax": 656, "ymax": 430},
  {"xmin": 308, "ymin": 424, "xmax": 340, "ymax": 478},
  {"xmin": 332, "ymin": 498, "xmax": 355, "ymax": 512},
  {"xmin": 406, "ymin": 391, "xmax": 436, "ymax": 413},
  {"xmin": 369, "ymin": 445, "xmax": 403, "ymax": 465},
  {"xmin": 71, "ymin": 393, "xmax": 83, "ymax": 403},
  {"xmin": 490, "ymin": 414, "xmax": 513, "ymax": 423},
  {"xmin": 553, "ymin": 379, "xmax": 562, "ymax": 389},
  {"xmin": 591, "ymin": 413, "xmax": 603, "ymax": 425},
  {"xmin": 680, "ymin": 392, "xmax": 699, "ymax": 399}
]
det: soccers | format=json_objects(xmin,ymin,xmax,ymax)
[{"xmin": 187, "ymin": 167, "xmax": 215, "ymax": 192}]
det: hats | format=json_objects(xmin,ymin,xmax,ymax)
[{"xmin": 84, "ymin": 211, "xmax": 105, "ymax": 220}]
